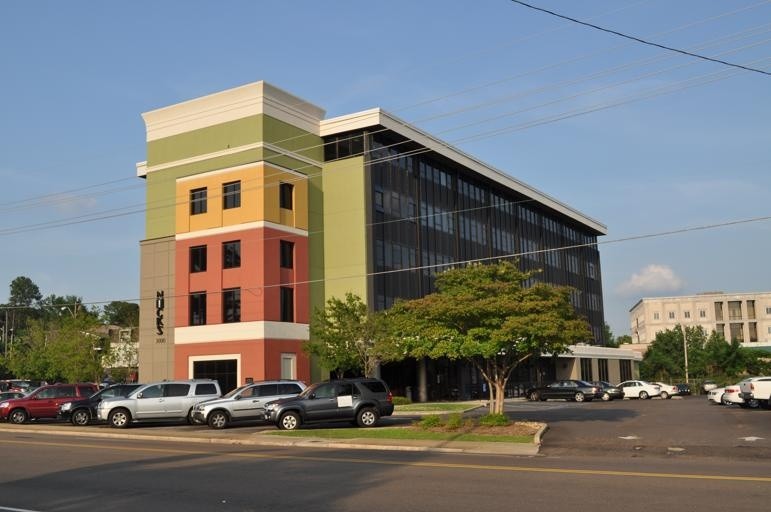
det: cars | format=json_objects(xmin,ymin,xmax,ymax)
[
  {"xmin": 527, "ymin": 379, "xmax": 691, "ymax": 402},
  {"xmin": 701, "ymin": 374, "xmax": 771, "ymax": 410}
]
